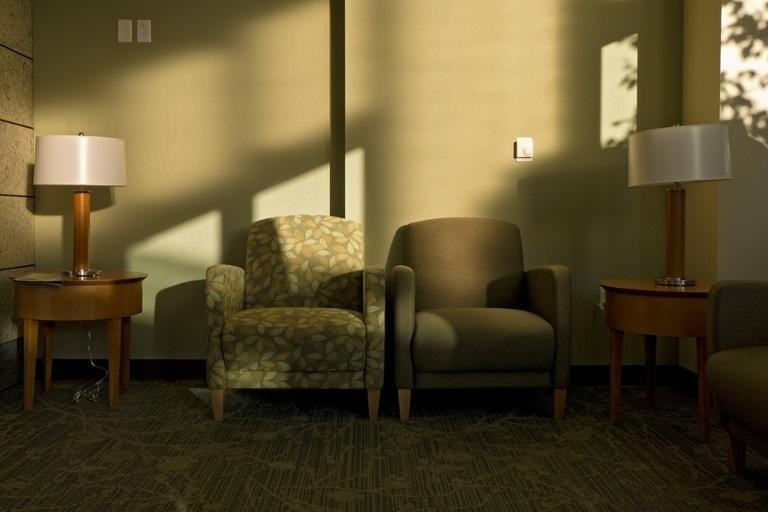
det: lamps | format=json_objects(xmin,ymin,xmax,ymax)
[
  {"xmin": 33, "ymin": 132, "xmax": 129, "ymax": 277},
  {"xmin": 624, "ymin": 121, "xmax": 734, "ymax": 286}
]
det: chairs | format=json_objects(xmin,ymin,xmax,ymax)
[{"xmin": 703, "ymin": 279, "xmax": 767, "ymax": 476}]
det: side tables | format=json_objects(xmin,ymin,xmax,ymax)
[
  {"xmin": 8, "ymin": 270, "xmax": 147, "ymax": 412},
  {"xmin": 595, "ymin": 277, "xmax": 725, "ymax": 443}
]
